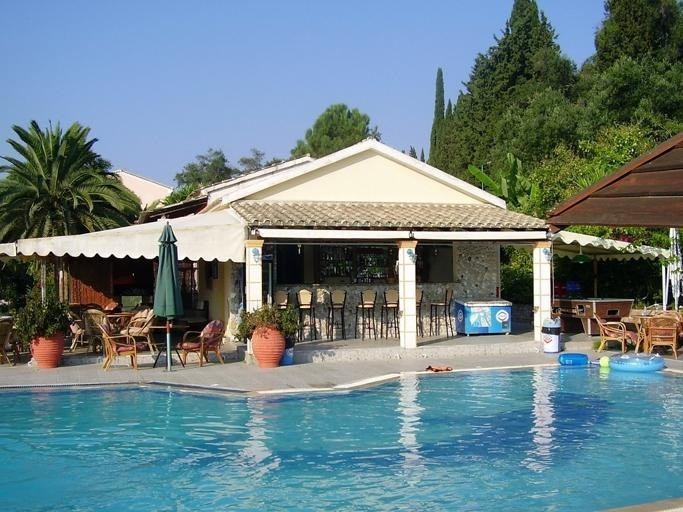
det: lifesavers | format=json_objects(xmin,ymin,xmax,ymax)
[{"xmin": 610, "ymin": 351, "xmax": 666, "ymax": 371}]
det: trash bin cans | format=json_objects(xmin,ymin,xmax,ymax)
[
  {"xmin": 542, "ymin": 319, "xmax": 561, "ymax": 353},
  {"xmin": 281, "ymin": 344, "xmax": 296, "ymax": 365}
]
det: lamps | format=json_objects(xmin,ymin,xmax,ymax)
[
  {"xmin": 541, "ymin": 248, "xmax": 552, "ymax": 262},
  {"xmin": 252, "ymin": 248, "xmax": 259, "ymax": 264},
  {"xmin": 407, "ymin": 249, "xmax": 417, "ymax": 265},
  {"xmin": 567, "ymin": 244, "xmax": 592, "ymax": 267}
]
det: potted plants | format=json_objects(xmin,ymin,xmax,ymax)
[
  {"xmin": 233, "ymin": 306, "xmax": 301, "ymax": 368},
  {"xmin": 13, "ymin": 299, "xmax": 72, "ymax": 368}
]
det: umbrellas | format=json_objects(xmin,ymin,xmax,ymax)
[
  {"xmin": 152, "ymin": 219, "xmax": 185, "ymax": 371},
  {"xmin": 668, "ymin": 227, "xmax": 681, "ymax": 310}
]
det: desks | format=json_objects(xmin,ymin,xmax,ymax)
[{"xmin": 150, "ymin": 325, "xmax": 187, "ymax": 367}]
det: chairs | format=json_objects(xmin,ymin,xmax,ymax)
[
  {"xmin": 643, "ymin": 316, "xmax": 678, "ymax": 359},
  {"xmin": 0, "ymin": 317, "xmax": 14, "ymax": 367},
  {"xmin": 66, "ymin": 311, "xmax": 96, "ymax": 352},
  {"xmin": 181, "ymin": 319, "xmax": 226, "ymax": 367},
  {"xmin": 273, "ymin": 288, "xmax": 454, "ymax": 339},
  {"xmin": 121, "ymin": 311, "xmax": 155, "ymax": 355},
  {"xmin": 80, "ymin": 308, "xmax": 114, "ymax": 353},
  {"xmin": 594, "ymin": 315, "xmax": 637, "ymax": 352},
  {"xmin": 96, "ymin": 323, "xmax": 137, "ymax": 370}
]
